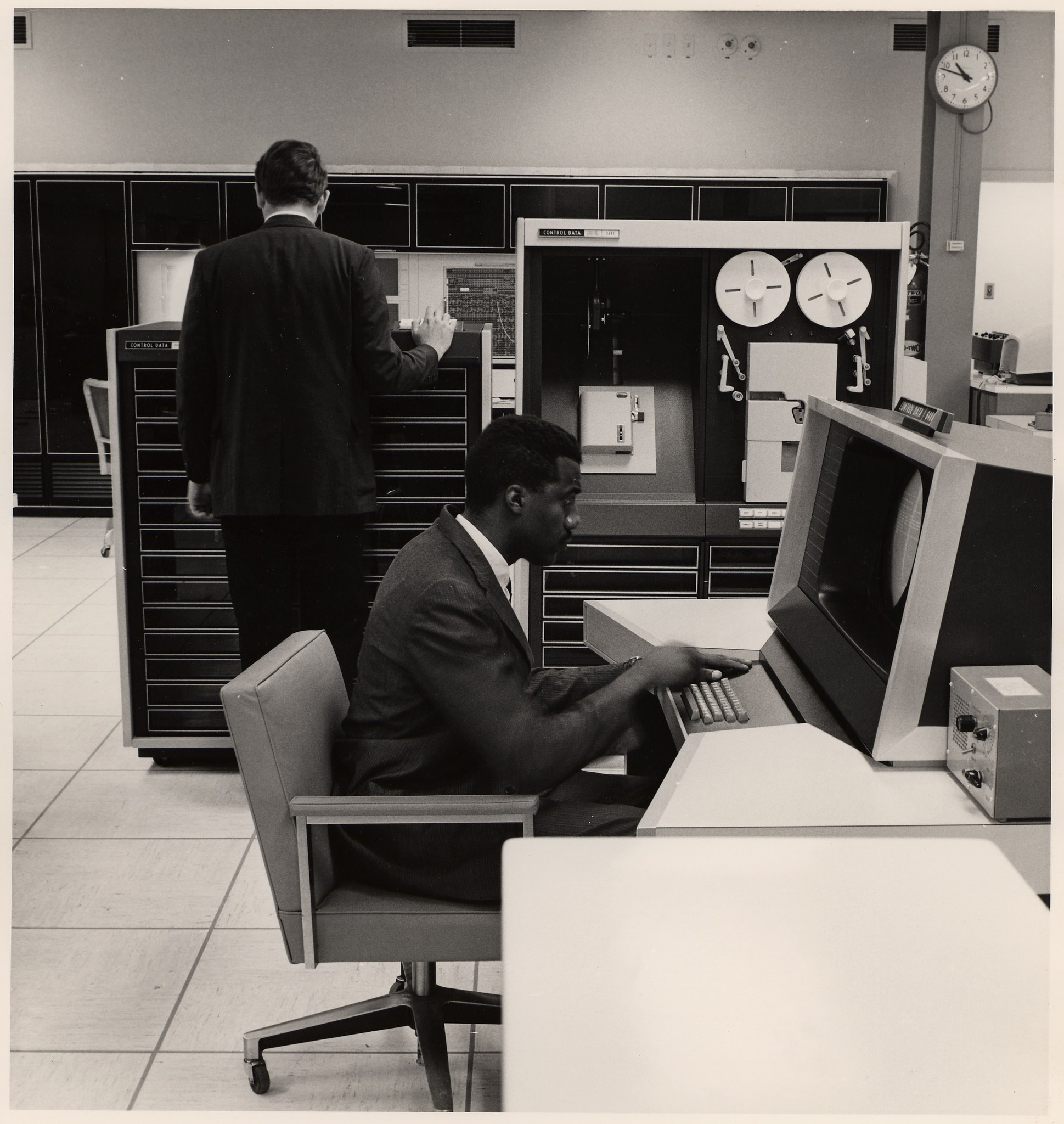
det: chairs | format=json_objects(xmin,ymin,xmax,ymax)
[
  {"xmin": 219, "ymin": 631, "xmax": 545, "ymax": 1112},
  {"xmin": 82, "ymin": 379, "xmax": 114, "ymax": 559}
]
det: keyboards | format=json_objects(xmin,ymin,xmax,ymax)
[{"xmin": 682, "ymin": 678, "xmax": 750, "ymax": 725}]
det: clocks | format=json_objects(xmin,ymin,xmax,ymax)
[{"xmin": 928, "ymin": 42, "xmax": 998, "ymax": 114}]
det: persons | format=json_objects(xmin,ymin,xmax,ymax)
[
  {"xmin": 326, "ymin": 413, "xmax": 756, "ymax": 913},
  {"xmin": 172, "ymin": 140, "xmax": 454, "ymax": 702}
]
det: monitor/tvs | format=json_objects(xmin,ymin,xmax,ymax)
[{"xmin": 768, "ymin": 395, "xmax": 1051, "ymax": 774}]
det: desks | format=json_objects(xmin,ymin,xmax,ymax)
[{"xmin": 969, "ymin": 370, "xmax": 1053, "ymax": 445}]
